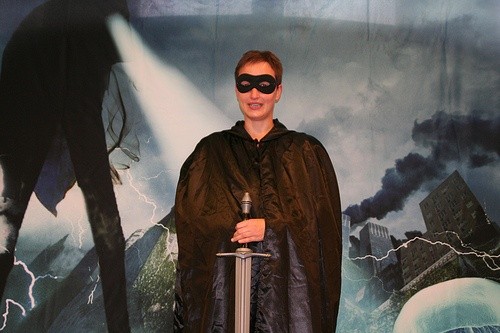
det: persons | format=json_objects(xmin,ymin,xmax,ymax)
[{"xmin": 173, "ymin": 49, "xmax": 343, "ymax": 332}]
